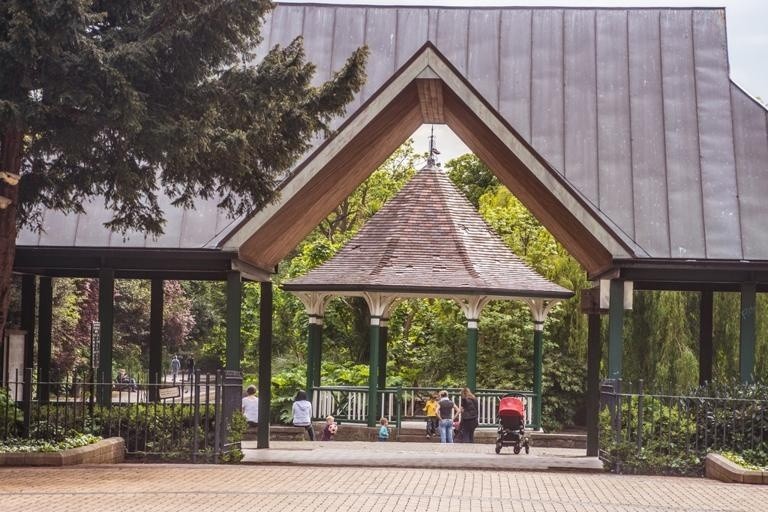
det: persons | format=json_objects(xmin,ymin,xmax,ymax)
[
  {"xmin": 320, "ymin": 415, "xmax": 338, "ymax": 440},
  {"xmin": 377, "ymin": 417, "xmax": 390, "ymax": 442},
  {"xmin": 187, "ymin": 353, "xmax": 196, "ymax": 382},
  {"xmin": 170, "ymin": 354, "xmax": 181, "ymax": 381},
  {"xmin": 292, "ymin": 389, "xmax": 315, "ymax": 440},
  {"xmin": 435, "ymin": 390, "xmax": 461, "ymax": 443},
  {"xmin": 241, "ymin": 384, "xmax": 258, "ymax": 427},
  {"xmin": 116, "ymin": 368, "xmax": 136, "ymax": 392},
  {"xmin": 421, "ymin": 393, "xmax": 439, "ymax": 438},
  {"xmin": 453, "ymin": 387, "xmax": 480, "ymax": 442}
]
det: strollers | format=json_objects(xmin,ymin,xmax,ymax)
[
  {"xmin": 494, "ymin": 394, "xmax": 530, "ymax": 454},
  {"xmin": 453, "ymin": 421, "xmax": 463, "ymax": 443}
]
